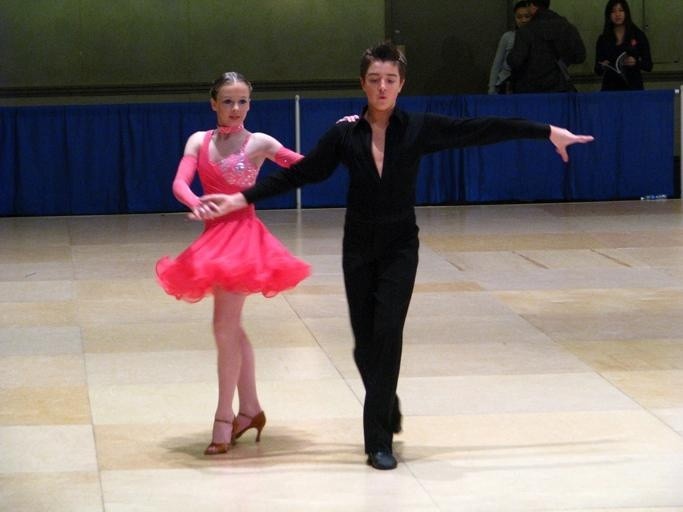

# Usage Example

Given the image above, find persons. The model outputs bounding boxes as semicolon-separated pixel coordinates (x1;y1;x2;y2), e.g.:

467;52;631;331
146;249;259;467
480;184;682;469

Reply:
487;1;530;97
496;0;586;94
186;37;598;471
592;0;654;91
153;70;364;455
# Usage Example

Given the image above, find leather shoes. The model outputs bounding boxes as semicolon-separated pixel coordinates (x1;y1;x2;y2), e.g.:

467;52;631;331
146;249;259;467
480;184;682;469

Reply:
370;450;396;469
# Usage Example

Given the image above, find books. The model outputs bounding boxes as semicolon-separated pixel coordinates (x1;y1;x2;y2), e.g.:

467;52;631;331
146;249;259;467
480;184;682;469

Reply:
598;51;628;74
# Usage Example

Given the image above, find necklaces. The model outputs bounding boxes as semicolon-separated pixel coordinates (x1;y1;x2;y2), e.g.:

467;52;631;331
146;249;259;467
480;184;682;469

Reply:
217;124;243;136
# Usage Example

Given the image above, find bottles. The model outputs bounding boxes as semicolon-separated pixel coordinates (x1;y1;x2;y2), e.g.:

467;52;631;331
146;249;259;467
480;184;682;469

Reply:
640;194;667;200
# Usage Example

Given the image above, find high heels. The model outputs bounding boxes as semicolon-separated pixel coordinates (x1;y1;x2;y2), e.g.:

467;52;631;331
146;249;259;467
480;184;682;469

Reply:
205;415;238;454
233;412;265;442
